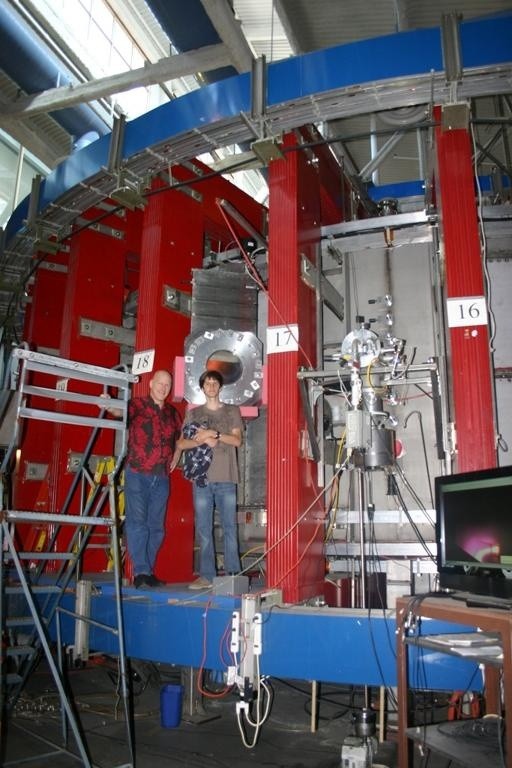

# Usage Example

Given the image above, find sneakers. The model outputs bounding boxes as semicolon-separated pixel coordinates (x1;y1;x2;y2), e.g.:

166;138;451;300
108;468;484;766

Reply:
189;577;213;590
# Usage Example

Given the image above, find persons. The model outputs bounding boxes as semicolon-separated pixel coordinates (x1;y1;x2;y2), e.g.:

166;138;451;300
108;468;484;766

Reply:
177;369;243;591
98;371;185;591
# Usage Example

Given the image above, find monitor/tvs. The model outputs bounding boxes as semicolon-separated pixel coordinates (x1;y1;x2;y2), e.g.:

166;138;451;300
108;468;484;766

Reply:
433;465;512;610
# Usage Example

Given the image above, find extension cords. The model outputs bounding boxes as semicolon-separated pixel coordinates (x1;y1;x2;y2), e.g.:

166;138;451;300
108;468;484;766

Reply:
231;612;239;652
252;613;261;654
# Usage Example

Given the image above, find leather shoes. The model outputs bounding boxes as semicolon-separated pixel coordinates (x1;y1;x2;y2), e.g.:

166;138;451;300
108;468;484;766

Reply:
135;575;152;589
148;574;167;587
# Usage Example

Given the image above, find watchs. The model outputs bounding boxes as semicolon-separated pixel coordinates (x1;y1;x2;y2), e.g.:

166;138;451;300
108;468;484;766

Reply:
214;429;222;440
